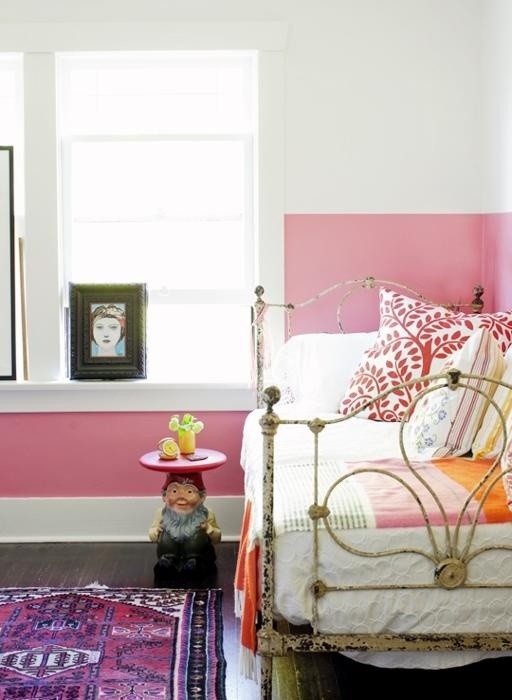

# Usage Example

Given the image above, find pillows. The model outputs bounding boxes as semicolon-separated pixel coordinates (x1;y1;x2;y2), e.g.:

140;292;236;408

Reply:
278;331;381;415
338;285;511;421
403;327;507;462
467;344;510;460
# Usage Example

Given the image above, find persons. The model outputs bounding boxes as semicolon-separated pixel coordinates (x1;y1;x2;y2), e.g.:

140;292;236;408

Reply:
148;473;222;589
90;305;125;357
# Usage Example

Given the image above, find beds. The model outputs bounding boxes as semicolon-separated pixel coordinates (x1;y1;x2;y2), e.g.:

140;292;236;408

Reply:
232;272;511;700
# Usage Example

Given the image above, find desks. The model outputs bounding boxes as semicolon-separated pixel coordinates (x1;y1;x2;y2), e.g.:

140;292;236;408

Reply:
137;446;228;578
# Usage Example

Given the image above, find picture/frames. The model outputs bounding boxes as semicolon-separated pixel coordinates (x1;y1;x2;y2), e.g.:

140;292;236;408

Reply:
65;280;148;383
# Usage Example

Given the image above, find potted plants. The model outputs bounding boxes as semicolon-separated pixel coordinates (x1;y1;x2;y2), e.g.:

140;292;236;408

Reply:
168;412;204;454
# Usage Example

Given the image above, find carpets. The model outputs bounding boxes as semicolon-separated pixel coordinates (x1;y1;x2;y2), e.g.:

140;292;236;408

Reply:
0;583;227;700
